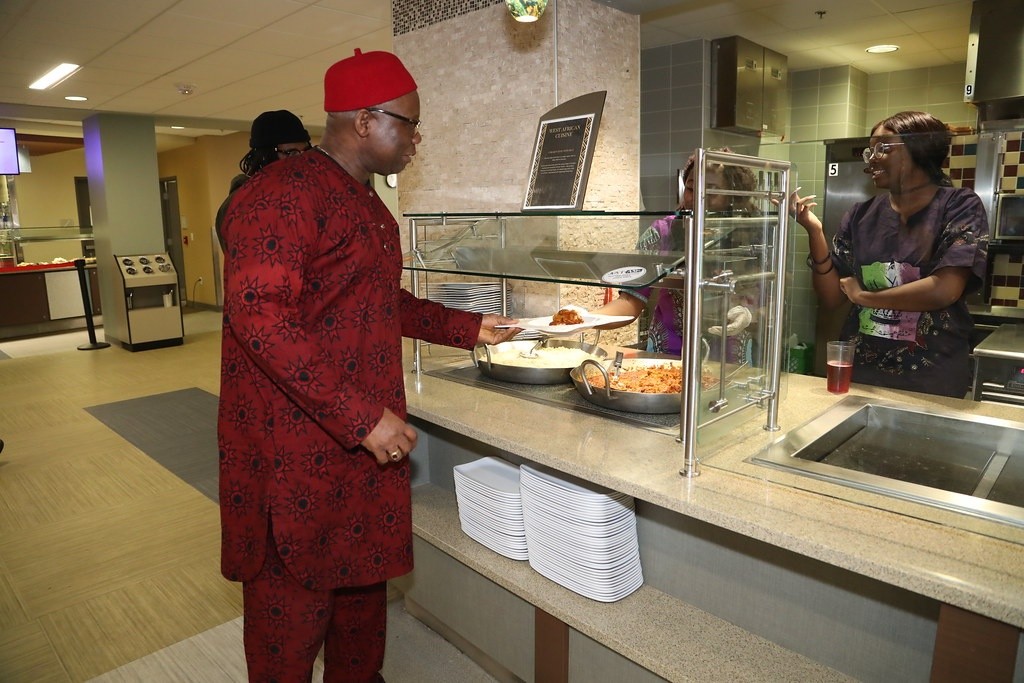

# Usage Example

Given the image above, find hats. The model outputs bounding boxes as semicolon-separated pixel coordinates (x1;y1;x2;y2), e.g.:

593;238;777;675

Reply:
250;110;311;149
323;48;417;113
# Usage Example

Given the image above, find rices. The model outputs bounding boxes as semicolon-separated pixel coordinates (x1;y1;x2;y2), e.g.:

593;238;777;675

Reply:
479;346;595;367
557;304;592;323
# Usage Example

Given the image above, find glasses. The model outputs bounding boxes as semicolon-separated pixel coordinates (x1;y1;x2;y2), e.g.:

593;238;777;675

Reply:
862;142;906;164
365;108;422;138
274;147;308;159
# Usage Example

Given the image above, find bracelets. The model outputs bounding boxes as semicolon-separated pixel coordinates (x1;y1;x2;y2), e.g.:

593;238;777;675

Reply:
806;253;834;274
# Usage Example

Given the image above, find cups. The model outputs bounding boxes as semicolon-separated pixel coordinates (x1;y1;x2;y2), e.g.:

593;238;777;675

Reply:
827;341;856;394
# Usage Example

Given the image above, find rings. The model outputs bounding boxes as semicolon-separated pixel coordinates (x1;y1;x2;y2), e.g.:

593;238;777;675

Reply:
392;449;401;460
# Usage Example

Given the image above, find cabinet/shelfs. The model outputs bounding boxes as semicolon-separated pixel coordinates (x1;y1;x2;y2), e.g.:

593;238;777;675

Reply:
712;34;788;139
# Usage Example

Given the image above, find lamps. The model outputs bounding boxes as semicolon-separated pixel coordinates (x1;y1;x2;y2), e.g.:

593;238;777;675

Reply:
505;0;548;22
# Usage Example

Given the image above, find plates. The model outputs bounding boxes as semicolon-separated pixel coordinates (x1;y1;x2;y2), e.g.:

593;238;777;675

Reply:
421;282;513;318
494;315;634;335
453;455;645;602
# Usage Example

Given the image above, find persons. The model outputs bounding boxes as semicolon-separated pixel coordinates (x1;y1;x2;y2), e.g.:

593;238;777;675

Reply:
771;111;989;399
589;147;774;366
217;48;526;683
215;110;313;251
